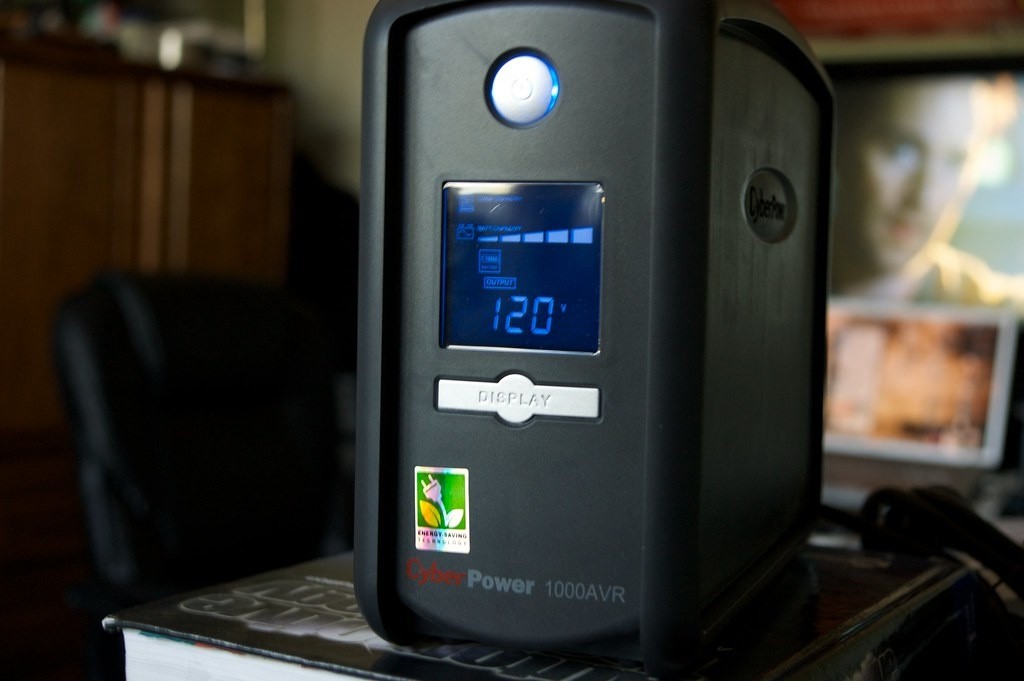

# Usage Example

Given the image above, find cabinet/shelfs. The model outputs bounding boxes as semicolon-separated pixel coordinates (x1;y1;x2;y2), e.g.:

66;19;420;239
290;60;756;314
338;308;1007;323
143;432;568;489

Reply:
0;14;295;681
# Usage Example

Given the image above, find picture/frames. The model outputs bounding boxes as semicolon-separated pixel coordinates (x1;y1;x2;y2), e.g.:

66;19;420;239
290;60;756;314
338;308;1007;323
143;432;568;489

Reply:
822;295;1018;469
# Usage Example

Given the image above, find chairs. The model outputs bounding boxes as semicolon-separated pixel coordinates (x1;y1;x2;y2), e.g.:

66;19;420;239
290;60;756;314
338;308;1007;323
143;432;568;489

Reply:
62;270;335;602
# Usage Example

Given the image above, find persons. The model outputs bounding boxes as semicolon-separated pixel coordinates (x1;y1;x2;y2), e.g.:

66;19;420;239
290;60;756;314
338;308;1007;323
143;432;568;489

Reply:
833;78;1024;308
